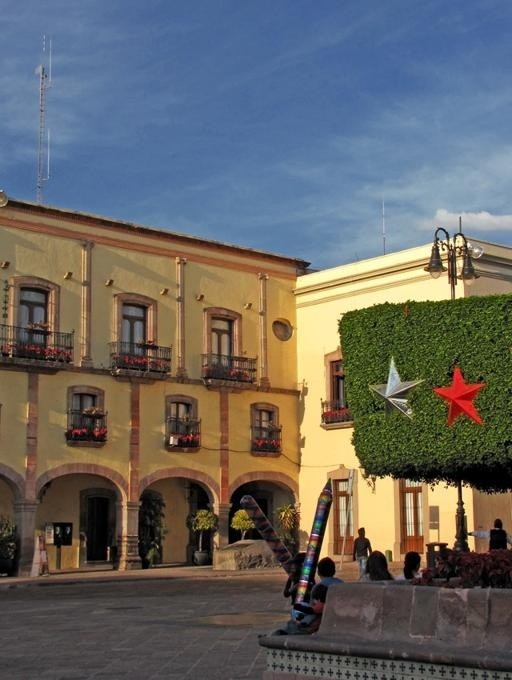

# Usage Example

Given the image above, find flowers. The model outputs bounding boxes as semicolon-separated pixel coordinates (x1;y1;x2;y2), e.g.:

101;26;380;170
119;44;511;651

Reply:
254;436;281;449
110;353;170;373
170;431;200;444
320;408;350;421
200;362;257;381
5;340;72;363
64;422;107;440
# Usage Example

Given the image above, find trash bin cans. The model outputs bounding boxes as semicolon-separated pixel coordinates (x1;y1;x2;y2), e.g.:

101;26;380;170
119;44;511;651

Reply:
107;546;116;562
426;542;448;568
386;550;392;561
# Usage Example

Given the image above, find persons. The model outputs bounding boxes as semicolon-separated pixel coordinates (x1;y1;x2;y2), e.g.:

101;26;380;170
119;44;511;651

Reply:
397;552;421;580
466;518;512;551
360;549;395;581
351;526;373;581
282;552;345;636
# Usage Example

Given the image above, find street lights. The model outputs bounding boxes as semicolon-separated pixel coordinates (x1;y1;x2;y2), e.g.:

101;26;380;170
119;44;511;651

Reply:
421;223;487;552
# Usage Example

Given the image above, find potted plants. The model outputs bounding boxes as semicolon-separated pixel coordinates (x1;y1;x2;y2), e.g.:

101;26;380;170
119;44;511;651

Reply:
190;507;218;565
